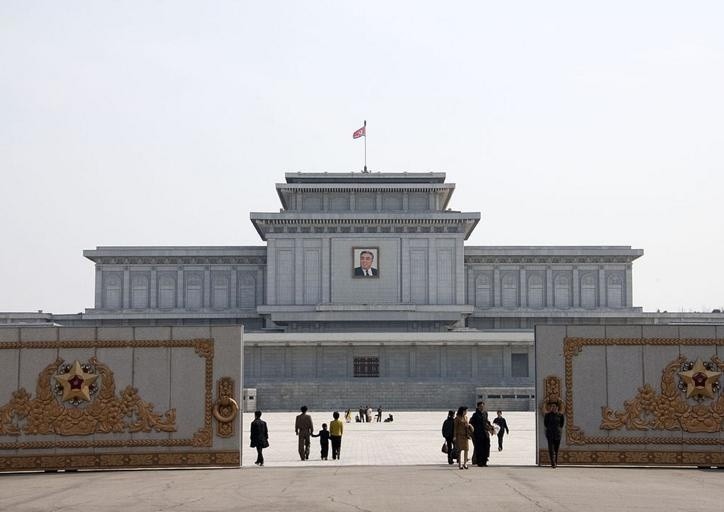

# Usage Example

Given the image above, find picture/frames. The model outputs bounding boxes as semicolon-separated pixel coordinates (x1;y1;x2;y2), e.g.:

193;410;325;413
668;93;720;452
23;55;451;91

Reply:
350;246;380;280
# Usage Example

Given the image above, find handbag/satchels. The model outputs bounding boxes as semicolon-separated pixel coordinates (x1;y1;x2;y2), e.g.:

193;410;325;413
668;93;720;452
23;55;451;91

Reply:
450;448;460;459
442;443;449;452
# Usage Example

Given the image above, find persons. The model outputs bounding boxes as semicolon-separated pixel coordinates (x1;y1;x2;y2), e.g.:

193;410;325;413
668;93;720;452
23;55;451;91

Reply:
355;251;377;277
295;406;313;460
250;411;269;466
544;402;564;468
312;424;332;460
330;412;343;459
442;402;508;469
353;127;365;139
345;405;393;423
